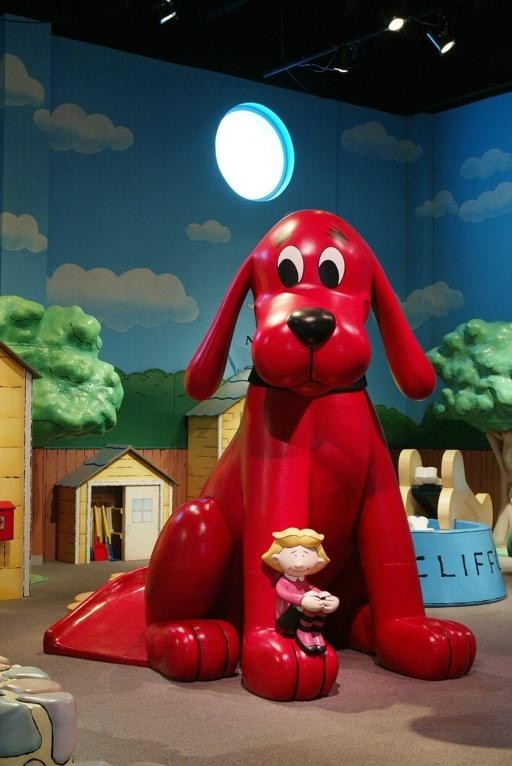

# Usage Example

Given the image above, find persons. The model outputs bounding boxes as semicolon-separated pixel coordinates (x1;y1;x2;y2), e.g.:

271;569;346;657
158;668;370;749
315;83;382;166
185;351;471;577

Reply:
260;526;339;655
